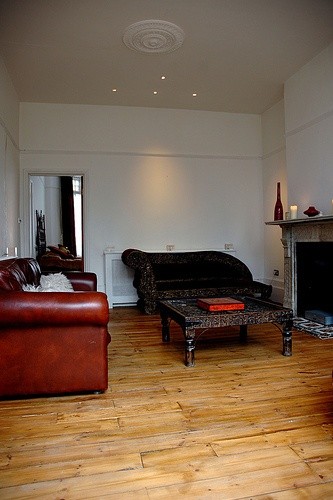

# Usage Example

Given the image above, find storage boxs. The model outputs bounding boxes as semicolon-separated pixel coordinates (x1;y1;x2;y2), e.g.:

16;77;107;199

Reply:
196;297;244;312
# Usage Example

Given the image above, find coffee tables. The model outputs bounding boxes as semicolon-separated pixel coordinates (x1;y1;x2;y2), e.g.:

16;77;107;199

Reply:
159;295;293;368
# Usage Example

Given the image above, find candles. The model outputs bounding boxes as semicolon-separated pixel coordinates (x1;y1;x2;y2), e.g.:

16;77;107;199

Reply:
290;205;298;219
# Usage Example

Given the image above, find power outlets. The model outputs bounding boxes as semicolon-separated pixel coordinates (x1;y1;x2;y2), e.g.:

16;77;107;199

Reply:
274;270;279;276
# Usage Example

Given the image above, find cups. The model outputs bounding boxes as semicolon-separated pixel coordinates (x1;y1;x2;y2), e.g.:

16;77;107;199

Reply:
290;205;298;220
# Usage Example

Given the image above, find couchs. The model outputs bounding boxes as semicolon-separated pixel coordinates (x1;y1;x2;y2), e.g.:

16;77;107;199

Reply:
120;248;272;315
0;243;111;400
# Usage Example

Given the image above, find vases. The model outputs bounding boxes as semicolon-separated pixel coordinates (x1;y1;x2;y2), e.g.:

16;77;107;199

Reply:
303;206;321;217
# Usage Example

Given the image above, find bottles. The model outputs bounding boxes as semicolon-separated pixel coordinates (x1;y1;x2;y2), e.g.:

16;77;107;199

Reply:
274;182;283;221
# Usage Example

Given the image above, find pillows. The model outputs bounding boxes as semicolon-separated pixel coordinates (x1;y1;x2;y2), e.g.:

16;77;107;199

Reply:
39;272;74;291
